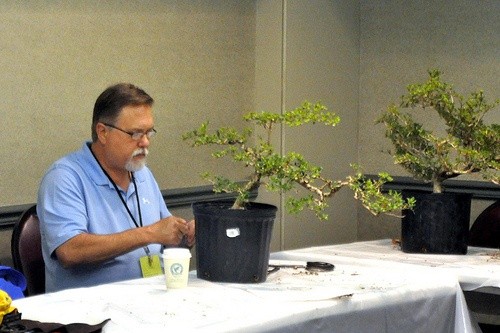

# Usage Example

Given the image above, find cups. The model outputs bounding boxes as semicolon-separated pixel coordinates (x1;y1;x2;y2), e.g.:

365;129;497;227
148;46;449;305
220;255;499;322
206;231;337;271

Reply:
160;248;192;289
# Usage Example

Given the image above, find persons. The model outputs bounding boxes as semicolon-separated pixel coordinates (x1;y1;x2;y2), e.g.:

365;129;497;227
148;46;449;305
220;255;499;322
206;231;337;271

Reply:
36;83;196;293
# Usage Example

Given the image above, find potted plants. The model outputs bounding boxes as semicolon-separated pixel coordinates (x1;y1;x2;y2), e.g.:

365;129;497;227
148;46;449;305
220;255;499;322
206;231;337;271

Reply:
182;98;419;283
374;66;500;255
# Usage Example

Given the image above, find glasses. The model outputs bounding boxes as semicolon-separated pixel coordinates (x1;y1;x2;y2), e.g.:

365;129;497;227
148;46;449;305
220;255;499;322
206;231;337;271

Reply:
103;122;157;141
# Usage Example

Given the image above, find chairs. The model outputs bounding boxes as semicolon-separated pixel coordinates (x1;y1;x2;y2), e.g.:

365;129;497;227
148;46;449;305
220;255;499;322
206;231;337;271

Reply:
10;204;46;298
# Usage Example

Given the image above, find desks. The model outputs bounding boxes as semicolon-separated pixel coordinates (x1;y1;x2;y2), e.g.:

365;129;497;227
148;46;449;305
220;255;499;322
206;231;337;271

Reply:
11;238;500;333
